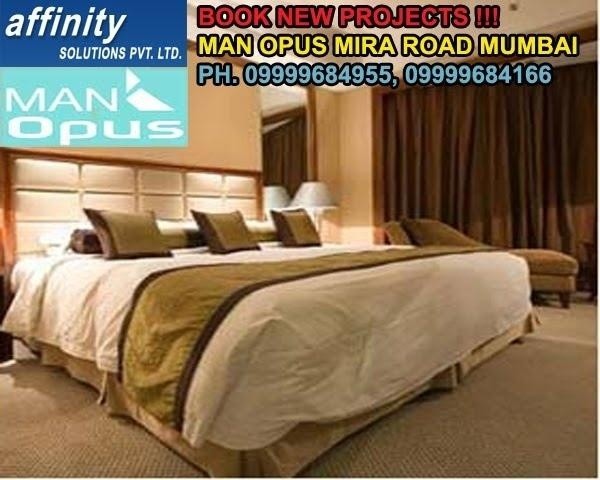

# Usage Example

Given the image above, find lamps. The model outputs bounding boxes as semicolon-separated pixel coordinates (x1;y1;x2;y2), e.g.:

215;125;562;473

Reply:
262;185;291;221
288;179;337;228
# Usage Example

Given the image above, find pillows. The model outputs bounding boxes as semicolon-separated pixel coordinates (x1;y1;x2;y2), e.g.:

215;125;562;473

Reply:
83;205;172;260
190;208;261;254
70;222;281;254
272;209;322;247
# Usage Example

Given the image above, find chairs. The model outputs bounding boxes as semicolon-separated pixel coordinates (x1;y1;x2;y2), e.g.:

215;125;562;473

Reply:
373;217;579;310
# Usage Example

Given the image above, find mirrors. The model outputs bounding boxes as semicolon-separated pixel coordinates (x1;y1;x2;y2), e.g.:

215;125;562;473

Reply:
258;79;321;222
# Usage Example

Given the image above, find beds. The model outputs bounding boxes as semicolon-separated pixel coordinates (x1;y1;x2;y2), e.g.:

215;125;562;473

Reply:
0;148;548;476
